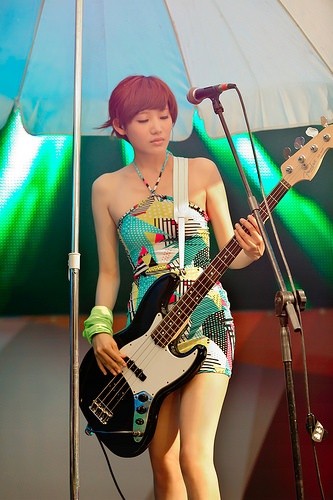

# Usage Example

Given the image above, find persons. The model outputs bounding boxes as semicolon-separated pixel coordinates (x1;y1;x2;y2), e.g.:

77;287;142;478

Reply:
80;75;266;500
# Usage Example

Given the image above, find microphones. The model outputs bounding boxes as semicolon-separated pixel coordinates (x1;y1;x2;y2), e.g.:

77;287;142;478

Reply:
186;83;236;105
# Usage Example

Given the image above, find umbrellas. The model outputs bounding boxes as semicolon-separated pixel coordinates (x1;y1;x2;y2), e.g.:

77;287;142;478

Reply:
1;0;333;500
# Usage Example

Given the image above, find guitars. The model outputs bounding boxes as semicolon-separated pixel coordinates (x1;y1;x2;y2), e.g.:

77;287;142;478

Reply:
78;115;333;458
